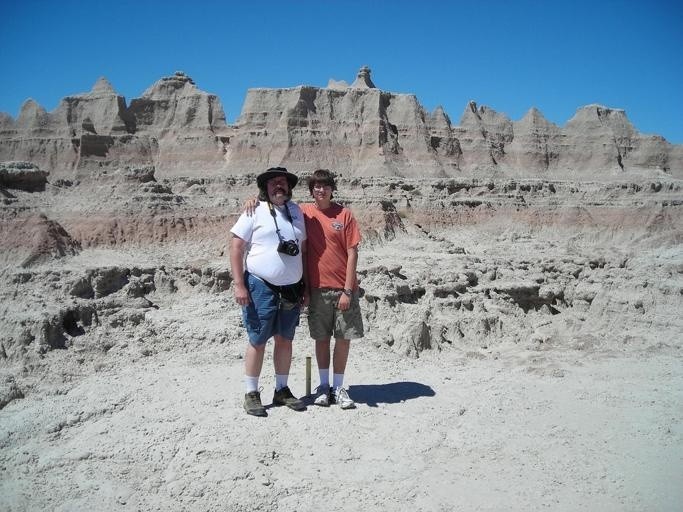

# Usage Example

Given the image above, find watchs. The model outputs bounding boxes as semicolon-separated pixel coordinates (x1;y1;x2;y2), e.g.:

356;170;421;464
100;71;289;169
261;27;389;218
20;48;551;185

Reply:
342;287;353;295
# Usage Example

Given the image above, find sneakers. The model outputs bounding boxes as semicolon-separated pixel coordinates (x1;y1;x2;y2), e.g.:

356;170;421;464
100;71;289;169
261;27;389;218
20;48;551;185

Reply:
243;391;266;415
273;385;306;410
313;384;330;406
330;386;355;409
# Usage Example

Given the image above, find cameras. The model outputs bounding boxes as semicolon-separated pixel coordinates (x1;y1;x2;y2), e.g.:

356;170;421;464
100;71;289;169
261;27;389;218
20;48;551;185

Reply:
277;239;300;255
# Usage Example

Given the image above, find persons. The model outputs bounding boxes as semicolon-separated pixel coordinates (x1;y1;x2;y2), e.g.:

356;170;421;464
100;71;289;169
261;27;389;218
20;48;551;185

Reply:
242;168;363;410
228;168;310;415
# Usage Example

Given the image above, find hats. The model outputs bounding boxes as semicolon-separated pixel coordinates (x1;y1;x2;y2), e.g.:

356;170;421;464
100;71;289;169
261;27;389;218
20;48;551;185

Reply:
257;167;298;191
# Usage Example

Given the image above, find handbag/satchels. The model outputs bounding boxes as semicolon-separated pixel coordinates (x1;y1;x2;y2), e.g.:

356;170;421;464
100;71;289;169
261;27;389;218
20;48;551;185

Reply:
258;274;306;304
